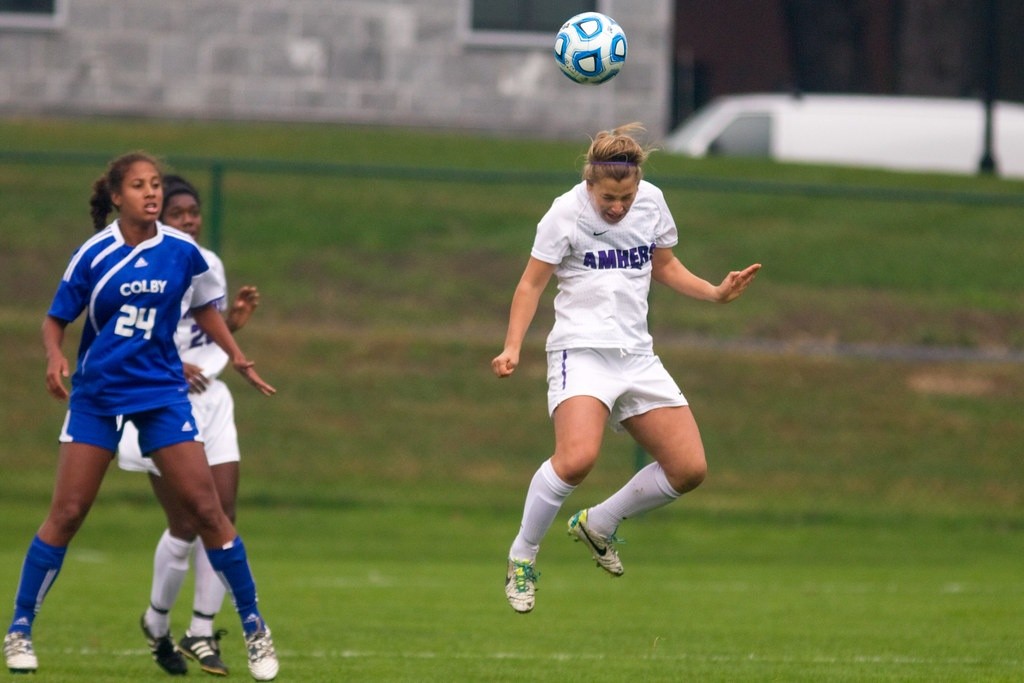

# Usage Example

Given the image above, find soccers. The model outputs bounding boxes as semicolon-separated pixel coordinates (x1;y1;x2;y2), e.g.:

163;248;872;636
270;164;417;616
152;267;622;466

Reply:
555;12;628;86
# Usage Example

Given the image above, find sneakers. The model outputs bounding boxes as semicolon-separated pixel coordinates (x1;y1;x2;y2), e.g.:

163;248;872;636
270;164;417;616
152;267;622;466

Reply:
177;631;228;676
140;614;188;675
3;631;39;673
506;556;536;612
239;621;280;680
567;507;625;575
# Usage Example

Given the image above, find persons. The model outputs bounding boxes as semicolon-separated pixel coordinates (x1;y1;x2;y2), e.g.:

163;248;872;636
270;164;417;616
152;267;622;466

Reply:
492;119;762;613
2;152;280;681
140;173;261;678
